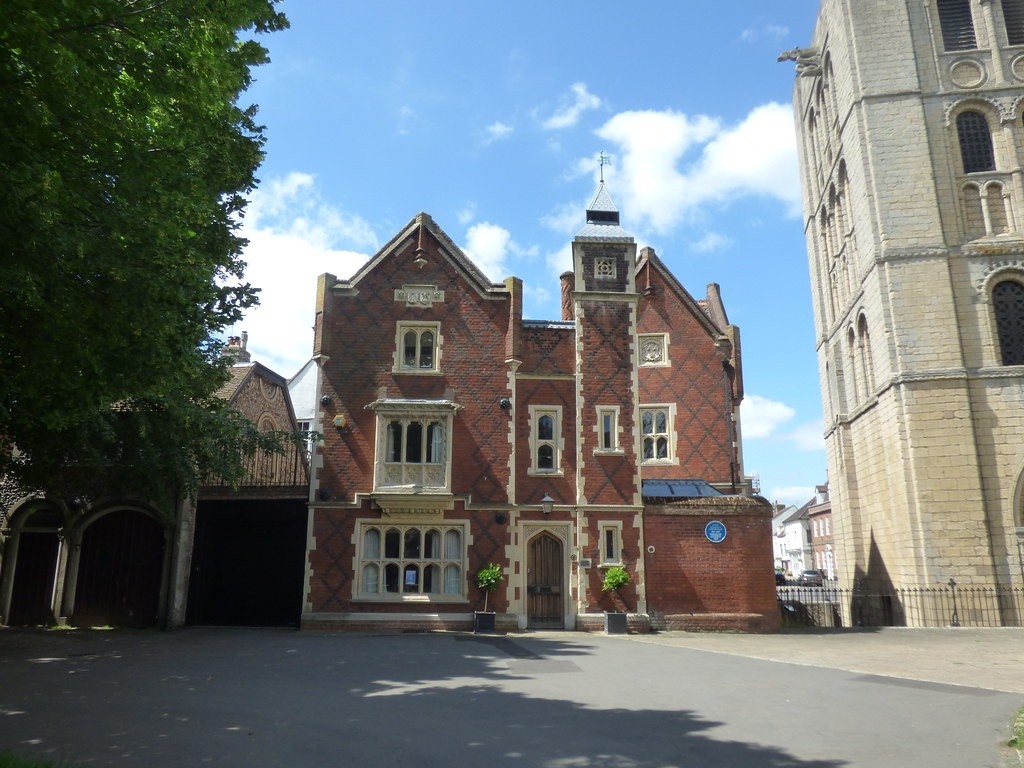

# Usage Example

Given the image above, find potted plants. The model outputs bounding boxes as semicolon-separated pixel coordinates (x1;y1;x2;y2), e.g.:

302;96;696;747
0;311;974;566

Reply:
471;561;504;632
600;566;631;635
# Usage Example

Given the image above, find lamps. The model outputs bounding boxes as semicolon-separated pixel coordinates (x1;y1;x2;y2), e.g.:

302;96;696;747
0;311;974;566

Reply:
540;492;555;517
333;415;347;429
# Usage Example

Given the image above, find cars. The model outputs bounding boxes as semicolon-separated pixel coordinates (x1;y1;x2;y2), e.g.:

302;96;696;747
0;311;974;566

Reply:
797;571;823;588
775;569;788;586
